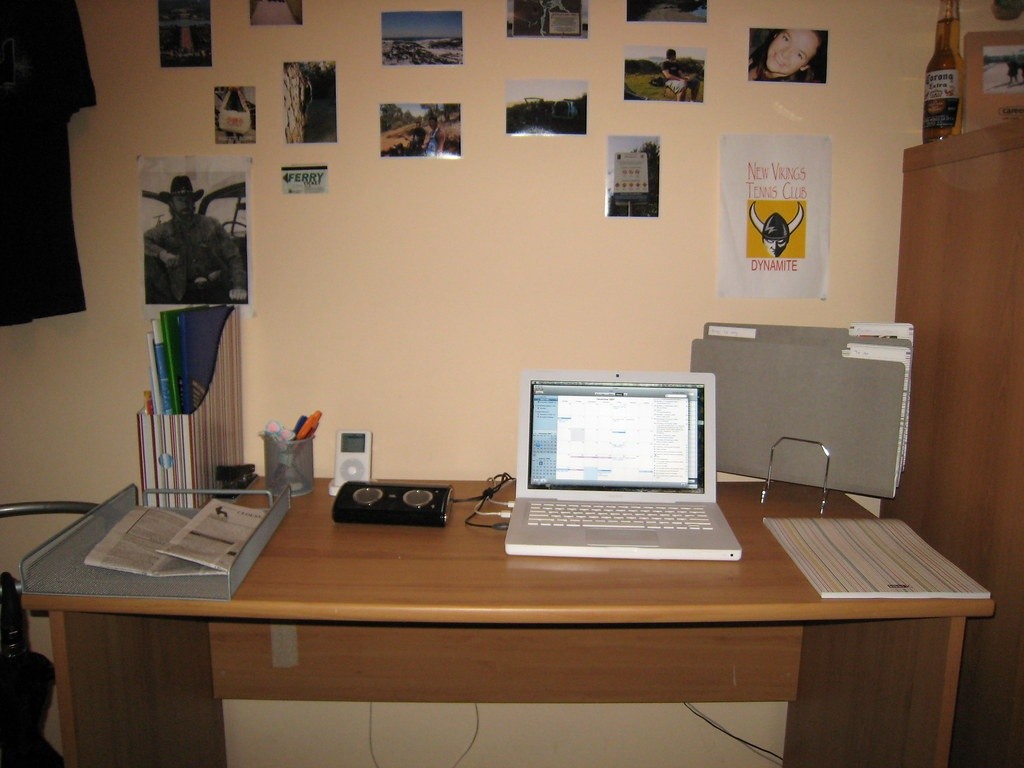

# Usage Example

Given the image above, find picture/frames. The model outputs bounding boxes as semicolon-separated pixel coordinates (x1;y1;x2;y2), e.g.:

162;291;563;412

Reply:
963;30;1024;134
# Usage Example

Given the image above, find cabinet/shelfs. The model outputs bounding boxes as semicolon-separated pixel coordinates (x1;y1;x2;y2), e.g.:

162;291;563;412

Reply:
879;117;1024;768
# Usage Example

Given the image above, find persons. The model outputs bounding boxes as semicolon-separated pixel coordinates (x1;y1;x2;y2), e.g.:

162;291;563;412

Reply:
748;28;821;82
412;115;445;157
142;175;248;310
661;48;700;102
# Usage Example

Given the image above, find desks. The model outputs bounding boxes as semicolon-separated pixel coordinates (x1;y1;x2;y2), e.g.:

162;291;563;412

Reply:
22;478;995;768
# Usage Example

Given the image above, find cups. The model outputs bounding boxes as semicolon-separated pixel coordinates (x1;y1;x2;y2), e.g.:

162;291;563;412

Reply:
264;440;314;495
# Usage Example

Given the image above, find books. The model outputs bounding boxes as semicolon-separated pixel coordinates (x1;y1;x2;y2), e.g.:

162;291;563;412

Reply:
143;304;234;416
762;517;991;599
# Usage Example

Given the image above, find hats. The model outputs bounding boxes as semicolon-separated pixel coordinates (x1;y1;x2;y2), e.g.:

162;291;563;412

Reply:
158;175;205;203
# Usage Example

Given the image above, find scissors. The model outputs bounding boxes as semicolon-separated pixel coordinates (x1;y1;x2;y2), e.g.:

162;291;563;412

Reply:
257;410;323;497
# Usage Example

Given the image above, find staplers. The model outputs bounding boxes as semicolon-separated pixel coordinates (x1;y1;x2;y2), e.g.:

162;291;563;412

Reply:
211;463;259;504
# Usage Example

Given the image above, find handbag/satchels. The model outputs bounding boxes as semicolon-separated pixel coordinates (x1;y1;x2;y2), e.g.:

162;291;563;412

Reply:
0;570;64;768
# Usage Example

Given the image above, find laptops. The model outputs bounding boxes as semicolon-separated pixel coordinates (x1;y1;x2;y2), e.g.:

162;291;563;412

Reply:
505;369;743;561
